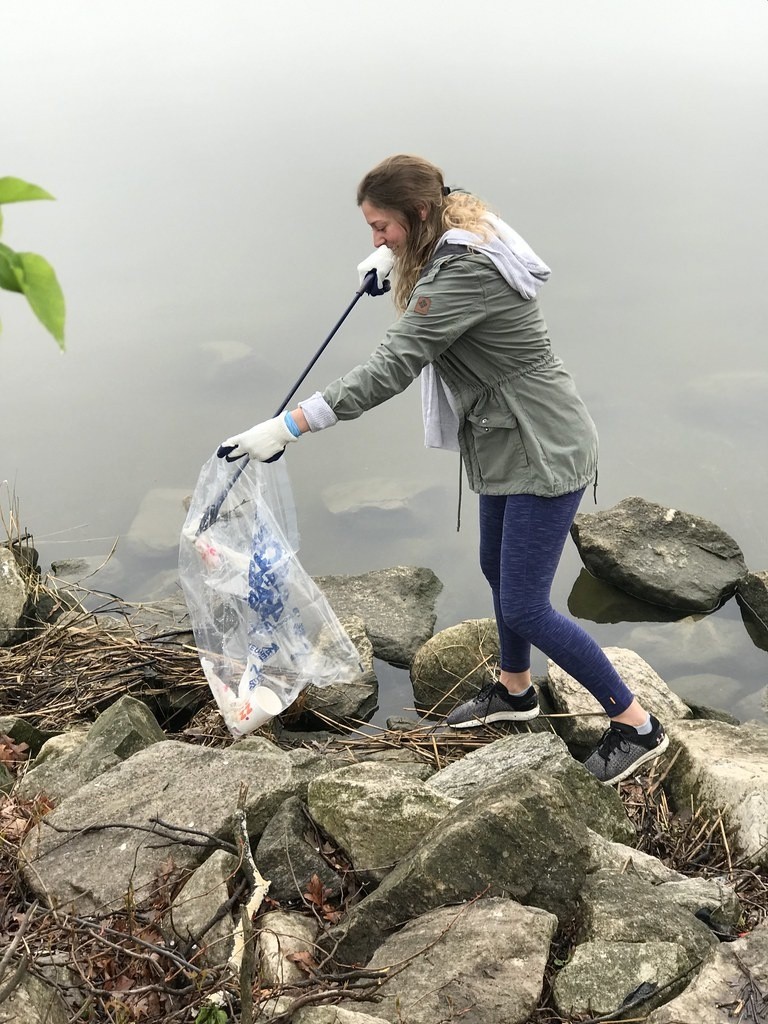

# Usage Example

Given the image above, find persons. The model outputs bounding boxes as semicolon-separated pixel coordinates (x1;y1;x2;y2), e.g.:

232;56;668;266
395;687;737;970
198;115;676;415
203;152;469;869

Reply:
217;154;669;788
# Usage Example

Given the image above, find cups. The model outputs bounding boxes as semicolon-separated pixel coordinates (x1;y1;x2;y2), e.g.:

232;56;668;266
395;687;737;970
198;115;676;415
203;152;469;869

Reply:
233;685;283;734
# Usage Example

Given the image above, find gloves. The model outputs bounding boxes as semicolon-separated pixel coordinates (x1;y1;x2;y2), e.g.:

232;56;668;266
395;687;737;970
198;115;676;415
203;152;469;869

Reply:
217;411;301;463
357;245;397;297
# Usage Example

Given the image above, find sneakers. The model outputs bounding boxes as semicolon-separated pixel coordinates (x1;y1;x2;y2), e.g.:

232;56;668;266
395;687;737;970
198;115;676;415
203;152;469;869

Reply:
446;682;540;728
584;714;670;786
